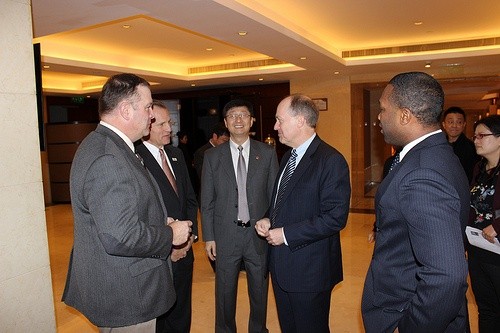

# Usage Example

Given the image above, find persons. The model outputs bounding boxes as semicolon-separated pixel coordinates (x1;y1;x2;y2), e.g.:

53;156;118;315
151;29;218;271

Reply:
134;99;198;333
360;72;469;333
367;145;403;243
442;107;481;185
464;115;500;333
176;131;191;177
255;94;350;333
201;100;279;333
61;74;192;333
194;124;231;271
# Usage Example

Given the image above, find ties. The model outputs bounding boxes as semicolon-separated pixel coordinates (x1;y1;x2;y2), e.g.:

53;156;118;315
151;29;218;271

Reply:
134;154;144;167
272;149;298;227
388;151;400;171
159;148;179;193
236;145;252;224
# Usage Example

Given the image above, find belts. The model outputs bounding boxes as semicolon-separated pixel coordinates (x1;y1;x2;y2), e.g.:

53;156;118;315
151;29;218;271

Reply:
237;220;250;227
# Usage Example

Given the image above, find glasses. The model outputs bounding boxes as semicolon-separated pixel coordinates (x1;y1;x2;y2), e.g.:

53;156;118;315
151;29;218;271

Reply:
473;133;495;140
224;113;251;120
